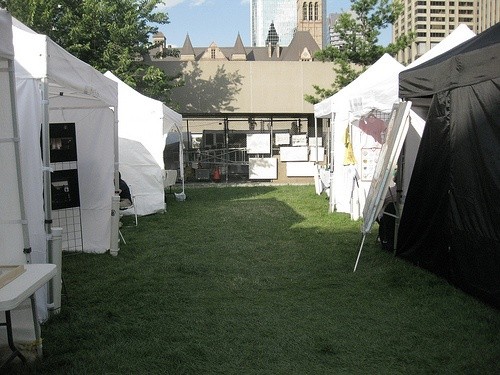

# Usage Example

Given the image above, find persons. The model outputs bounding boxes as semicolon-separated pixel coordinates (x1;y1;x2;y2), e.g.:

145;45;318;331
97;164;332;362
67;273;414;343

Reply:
113;170;133;227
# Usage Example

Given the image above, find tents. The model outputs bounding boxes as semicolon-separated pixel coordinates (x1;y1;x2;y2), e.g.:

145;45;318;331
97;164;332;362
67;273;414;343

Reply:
102;70;186;216
314;52;406;213
403;24;477;72
12;16;122;315
0;6;43;366
379;22;500;313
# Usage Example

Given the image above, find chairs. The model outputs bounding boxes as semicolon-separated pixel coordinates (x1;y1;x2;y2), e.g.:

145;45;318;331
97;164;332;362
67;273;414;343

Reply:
119;184;137;226
166;170;177;194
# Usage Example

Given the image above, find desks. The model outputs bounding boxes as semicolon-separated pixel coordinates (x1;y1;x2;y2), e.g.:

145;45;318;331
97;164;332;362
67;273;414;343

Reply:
0;264;57;375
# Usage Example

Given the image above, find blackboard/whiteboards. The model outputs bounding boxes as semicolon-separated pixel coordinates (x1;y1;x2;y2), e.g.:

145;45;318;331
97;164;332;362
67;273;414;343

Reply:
246;133;325;181
